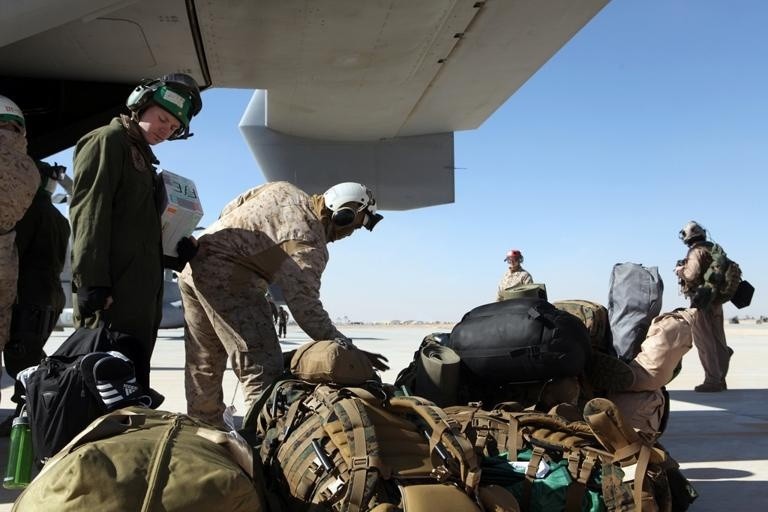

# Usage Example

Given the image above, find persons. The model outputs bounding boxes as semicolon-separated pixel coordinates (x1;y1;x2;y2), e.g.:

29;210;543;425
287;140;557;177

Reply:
5;160;72;413
672;220;755;390
72;75;202;384
178;181;389;430
278;307;289;338
496;251;534;302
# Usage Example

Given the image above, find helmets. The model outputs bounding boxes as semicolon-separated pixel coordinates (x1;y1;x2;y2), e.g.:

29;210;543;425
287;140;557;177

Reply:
0;95;25;125
34;162;57;195
679;220;705;246
325;183;382;231
126;75;202;140
504;249;523;263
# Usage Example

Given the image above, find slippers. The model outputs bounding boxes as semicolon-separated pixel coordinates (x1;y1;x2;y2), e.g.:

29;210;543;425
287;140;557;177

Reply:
93;357;139;413
81;352;114;412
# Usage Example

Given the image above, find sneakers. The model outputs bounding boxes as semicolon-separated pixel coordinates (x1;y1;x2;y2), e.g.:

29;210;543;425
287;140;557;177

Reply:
695;380;726;392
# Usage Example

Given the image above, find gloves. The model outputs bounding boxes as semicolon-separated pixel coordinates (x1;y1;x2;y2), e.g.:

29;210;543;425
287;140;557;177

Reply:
77;288;112;316
175;236;199;262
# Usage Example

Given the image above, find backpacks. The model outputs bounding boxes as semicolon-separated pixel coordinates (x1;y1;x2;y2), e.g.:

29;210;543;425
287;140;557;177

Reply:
0;93;41;353
289;339;385;388
689;243;743;306
14;408;268;511
442;396;699;512
393;297;593;412
241;375;483;512
16;306;166;471
